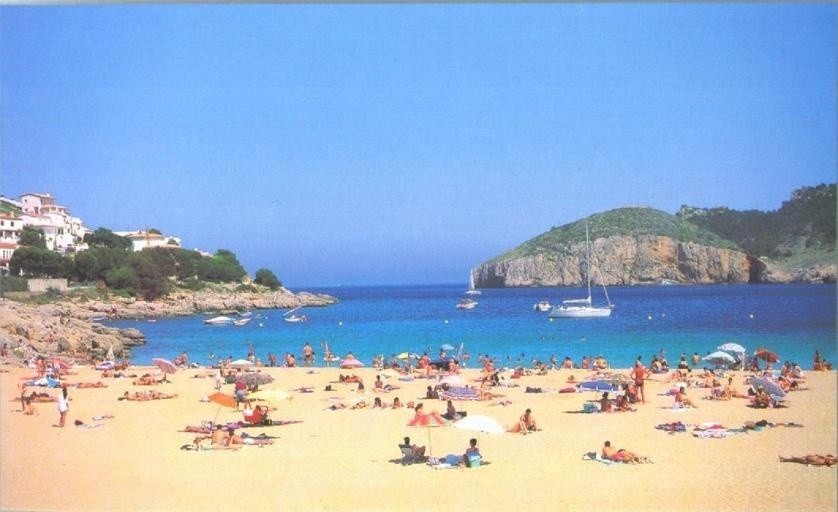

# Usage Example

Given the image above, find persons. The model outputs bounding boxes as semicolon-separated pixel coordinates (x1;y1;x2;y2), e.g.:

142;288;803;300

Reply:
104;304;117;314
0;338;837;468
59;315;72;328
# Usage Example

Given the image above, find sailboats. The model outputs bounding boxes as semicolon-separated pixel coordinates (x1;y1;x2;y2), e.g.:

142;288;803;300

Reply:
548;222;615;317
466;269;482;296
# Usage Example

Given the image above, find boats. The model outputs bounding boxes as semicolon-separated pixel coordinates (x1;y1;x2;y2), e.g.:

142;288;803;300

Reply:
532;301;550;312
455;297;477;309
286;315;307;323
204;316;249;326
